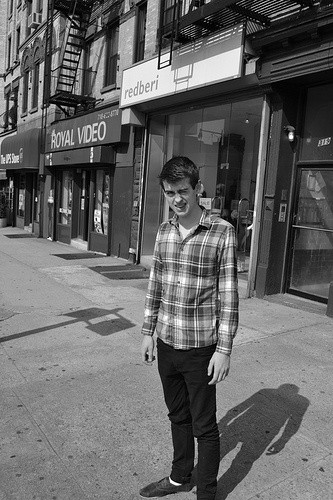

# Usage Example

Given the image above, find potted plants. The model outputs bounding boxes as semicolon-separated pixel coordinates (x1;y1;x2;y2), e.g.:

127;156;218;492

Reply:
0;194;7;227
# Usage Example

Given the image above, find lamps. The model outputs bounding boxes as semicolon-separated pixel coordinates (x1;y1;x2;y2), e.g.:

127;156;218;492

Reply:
284;126;295;142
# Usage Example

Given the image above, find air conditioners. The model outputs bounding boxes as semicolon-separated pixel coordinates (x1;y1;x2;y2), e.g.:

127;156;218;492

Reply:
28;13;42;28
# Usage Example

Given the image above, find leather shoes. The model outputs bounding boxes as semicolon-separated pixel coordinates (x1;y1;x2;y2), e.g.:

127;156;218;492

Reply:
139;475;192;497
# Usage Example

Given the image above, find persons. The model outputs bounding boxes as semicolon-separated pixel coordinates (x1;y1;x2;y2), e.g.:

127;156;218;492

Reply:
140;155;239;500
